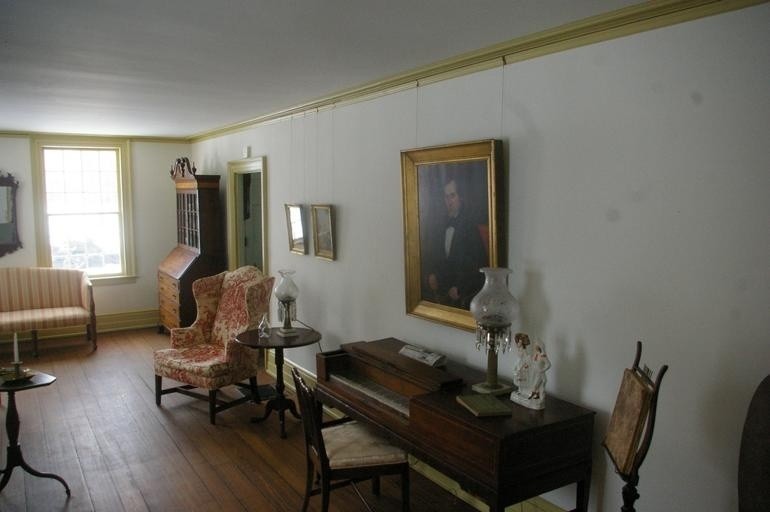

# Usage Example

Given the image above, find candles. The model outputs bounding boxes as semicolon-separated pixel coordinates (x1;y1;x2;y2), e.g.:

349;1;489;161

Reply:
13;332;19;362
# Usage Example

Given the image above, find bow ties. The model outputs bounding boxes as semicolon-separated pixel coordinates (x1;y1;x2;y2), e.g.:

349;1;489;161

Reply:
446;215;456;227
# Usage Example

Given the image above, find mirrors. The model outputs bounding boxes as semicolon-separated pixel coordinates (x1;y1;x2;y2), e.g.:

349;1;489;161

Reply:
0;169;23;257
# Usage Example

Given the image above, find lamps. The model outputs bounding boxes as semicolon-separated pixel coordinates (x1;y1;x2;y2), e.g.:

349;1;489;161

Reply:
470;267;520;396
274;268;299;338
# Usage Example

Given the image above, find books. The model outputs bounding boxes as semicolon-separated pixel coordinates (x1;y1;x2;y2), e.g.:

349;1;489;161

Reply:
604;368;656;476
456;393;512;417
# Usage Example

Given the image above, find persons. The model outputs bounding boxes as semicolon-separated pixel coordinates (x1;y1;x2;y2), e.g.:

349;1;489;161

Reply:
427;176;489;311
512;337;551;400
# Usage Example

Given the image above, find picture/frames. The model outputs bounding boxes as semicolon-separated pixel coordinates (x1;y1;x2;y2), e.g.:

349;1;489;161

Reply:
400;138;499;334
284;203;308;256
310;203;335;262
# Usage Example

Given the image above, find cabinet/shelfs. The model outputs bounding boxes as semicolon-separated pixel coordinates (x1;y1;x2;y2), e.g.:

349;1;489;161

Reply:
157;156;221;333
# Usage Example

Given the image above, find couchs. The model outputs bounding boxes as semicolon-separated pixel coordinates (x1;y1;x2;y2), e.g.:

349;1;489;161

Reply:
0;266;97;358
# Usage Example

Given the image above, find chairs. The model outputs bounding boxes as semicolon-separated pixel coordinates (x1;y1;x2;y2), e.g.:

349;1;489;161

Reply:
153;264;276;425
291;367;410;512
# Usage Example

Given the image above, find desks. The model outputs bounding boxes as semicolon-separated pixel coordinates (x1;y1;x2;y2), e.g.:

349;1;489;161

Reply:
0;365;71;498
235;327;321;439
